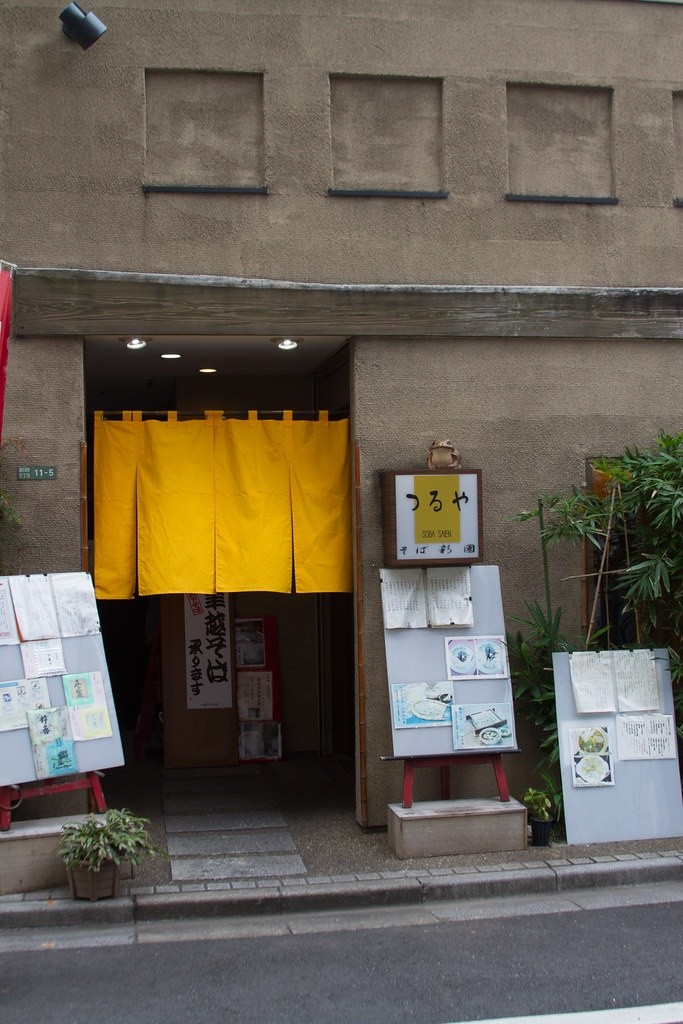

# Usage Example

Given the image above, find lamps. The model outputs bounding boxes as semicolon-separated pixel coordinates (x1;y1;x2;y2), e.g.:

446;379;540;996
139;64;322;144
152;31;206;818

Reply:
58;1;107;51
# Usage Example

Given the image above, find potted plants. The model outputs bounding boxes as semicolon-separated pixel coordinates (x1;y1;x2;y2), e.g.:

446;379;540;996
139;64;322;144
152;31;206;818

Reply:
525;787;554;845
59;809;170;899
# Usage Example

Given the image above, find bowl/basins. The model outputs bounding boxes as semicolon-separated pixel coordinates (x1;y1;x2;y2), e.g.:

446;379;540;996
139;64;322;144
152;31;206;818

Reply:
440;694;452;703
499;726;511;736
479;728;501;745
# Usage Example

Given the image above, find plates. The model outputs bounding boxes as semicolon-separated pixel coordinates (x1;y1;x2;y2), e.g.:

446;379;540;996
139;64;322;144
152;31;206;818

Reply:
577;728;608;755
450;644;476;674
476;642;503;675
576;755;608;782
